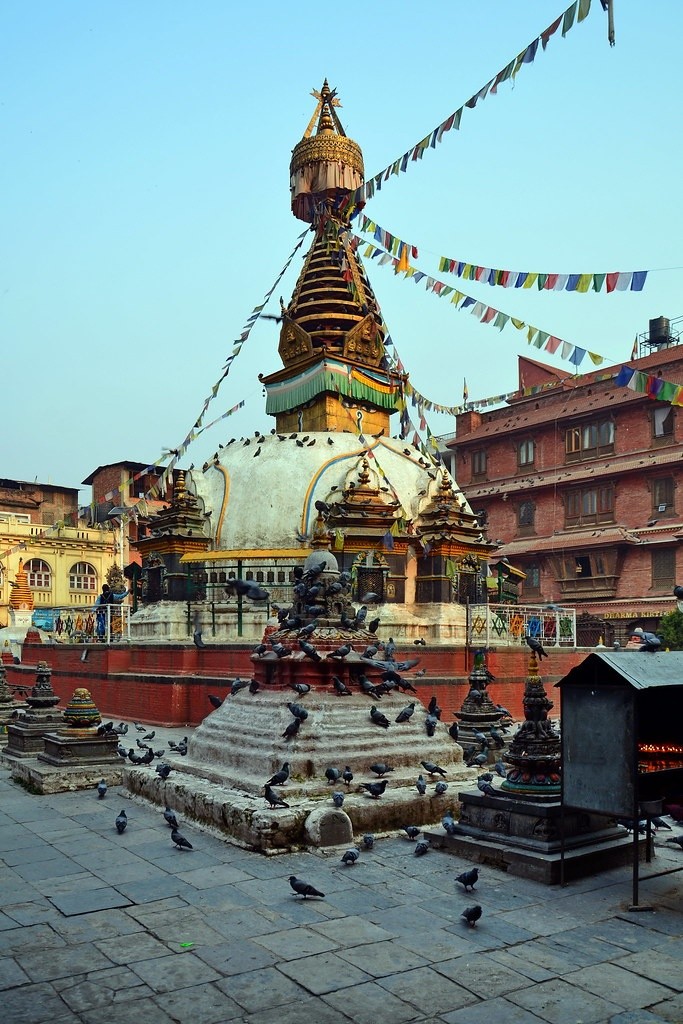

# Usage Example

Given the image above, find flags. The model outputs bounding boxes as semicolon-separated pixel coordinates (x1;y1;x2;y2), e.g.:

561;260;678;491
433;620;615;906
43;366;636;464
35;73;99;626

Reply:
631;338;638;360
464;382;468;399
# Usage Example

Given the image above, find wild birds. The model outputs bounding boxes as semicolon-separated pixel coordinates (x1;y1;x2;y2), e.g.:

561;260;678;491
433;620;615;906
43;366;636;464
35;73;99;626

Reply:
95;423;683;927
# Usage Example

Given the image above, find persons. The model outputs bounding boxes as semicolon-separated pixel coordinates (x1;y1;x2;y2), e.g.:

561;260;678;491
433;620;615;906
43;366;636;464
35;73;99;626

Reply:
92;584;131;641
